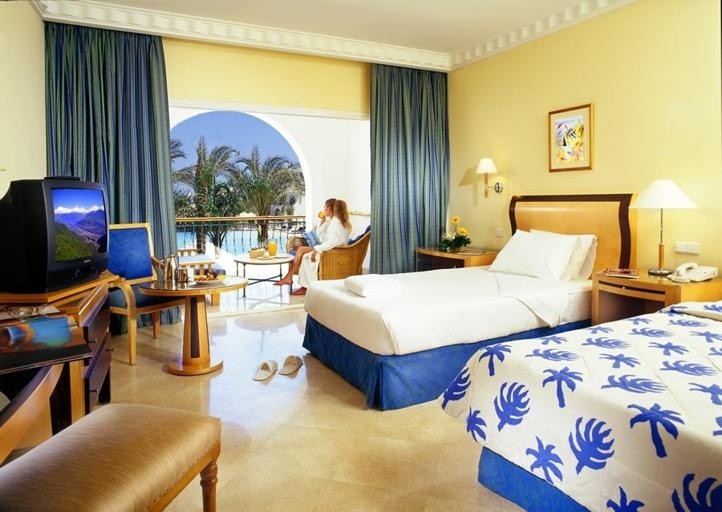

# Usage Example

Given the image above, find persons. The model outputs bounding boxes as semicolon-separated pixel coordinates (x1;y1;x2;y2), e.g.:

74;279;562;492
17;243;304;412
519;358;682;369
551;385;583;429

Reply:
274;199;350;295
290;198;336;274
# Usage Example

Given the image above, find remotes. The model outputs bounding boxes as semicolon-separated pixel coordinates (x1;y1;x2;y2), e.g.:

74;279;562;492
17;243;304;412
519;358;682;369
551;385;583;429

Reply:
38;175;86;182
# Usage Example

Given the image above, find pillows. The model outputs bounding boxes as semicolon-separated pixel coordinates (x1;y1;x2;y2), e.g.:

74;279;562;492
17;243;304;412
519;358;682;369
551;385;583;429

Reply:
488;227;597;284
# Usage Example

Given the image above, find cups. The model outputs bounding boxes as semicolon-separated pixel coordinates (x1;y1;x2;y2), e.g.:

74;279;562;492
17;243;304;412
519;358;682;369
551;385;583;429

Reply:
318;210;326;219
264;238;278;256
177;267;188;282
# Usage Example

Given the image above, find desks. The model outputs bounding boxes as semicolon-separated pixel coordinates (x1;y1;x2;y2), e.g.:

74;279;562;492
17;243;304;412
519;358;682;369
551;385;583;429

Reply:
0;362;64;468
415;245;501;272
591;270;722;326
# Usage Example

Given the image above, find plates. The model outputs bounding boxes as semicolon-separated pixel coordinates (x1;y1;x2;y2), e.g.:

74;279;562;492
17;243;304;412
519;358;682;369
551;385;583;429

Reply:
194;279;223;284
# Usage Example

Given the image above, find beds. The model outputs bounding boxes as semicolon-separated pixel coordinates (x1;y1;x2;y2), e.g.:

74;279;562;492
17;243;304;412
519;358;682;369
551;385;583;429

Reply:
304;192;638;411
476;301;722;512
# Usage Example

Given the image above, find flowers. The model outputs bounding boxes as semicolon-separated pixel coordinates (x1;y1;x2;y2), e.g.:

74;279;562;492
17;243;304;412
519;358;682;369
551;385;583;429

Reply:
440;215;472;253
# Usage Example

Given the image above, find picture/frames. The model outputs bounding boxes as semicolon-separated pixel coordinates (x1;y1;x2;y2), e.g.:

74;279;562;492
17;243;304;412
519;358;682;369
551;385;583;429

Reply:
547;102;594;173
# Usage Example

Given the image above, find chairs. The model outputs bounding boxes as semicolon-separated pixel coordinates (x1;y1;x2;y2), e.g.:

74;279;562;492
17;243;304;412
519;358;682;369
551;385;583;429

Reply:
286;228;373;280
106;222;185;365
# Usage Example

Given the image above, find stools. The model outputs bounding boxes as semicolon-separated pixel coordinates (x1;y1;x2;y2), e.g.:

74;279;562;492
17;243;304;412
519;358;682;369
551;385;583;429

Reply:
0;403;222;512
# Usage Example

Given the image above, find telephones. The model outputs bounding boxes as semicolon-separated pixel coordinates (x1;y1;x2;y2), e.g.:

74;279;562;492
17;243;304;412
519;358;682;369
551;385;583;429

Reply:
669;262;717;282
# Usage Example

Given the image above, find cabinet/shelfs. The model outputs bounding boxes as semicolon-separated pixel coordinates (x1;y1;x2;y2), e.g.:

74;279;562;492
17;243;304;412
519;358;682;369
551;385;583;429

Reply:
0;280;114;436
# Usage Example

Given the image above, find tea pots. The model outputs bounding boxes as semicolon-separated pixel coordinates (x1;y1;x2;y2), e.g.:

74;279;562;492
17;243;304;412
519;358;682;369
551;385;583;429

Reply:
158;255;178;282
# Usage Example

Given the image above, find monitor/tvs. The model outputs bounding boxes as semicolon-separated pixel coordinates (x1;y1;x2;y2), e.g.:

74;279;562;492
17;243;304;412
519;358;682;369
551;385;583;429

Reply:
0;179;111;294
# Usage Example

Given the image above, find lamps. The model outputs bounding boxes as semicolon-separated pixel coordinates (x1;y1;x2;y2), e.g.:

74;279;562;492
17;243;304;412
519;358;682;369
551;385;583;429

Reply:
627;177;696;277
475;157;504;199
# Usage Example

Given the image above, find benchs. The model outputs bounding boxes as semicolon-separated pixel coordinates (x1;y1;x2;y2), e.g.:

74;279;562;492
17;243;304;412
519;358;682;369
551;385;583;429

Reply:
176;248;226;307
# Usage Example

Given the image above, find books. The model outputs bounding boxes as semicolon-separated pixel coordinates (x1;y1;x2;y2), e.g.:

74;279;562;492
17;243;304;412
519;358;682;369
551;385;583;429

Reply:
603;267;640;279
0;305;94;376
457;249;484;255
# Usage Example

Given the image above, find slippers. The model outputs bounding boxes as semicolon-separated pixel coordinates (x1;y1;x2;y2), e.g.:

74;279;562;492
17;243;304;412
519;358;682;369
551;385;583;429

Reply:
253;359;278;381
278;355;302;375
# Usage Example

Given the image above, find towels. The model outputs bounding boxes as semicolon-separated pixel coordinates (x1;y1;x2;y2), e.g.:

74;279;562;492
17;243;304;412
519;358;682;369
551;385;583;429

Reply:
343;274;404;299
